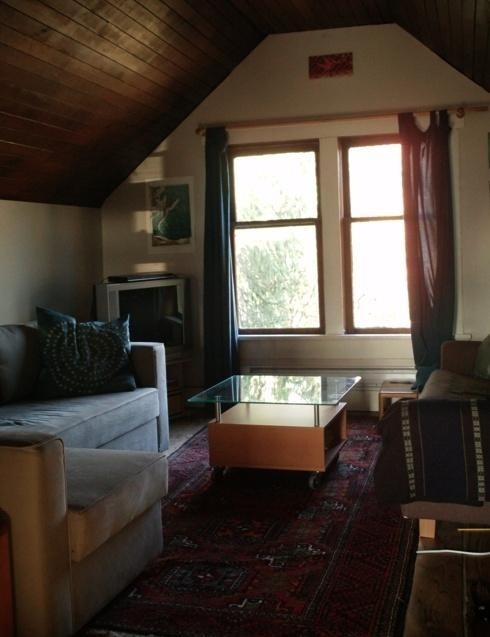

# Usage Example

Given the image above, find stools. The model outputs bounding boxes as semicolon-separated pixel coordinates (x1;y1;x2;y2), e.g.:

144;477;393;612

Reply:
378;380;418;421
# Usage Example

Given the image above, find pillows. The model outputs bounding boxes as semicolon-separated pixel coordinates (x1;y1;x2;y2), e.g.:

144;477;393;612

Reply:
16;305;138;397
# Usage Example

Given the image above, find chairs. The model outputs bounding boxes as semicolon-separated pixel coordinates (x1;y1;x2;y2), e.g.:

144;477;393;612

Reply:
392;335;490;541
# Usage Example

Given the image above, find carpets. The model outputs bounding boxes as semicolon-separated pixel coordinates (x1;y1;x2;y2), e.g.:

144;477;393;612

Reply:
83;407;418;636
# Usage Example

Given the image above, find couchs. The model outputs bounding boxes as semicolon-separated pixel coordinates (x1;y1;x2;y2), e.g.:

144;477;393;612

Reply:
1;313;172;637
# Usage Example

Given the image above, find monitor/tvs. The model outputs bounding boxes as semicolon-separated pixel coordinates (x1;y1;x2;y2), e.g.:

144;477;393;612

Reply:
92;279;187;363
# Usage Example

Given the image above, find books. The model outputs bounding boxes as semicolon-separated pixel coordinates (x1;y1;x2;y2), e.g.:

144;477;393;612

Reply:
108;274;176;283
